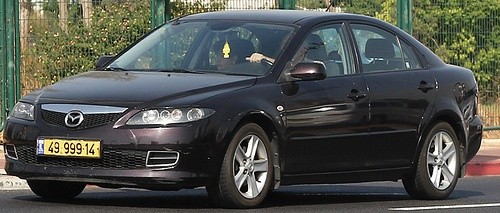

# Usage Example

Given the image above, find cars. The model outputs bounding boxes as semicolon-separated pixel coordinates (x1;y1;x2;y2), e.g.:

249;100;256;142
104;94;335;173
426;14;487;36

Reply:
3;10;483;209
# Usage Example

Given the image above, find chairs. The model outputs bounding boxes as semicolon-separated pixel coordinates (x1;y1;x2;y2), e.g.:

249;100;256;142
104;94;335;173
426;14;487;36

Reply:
230;39;255;64
306;33;342;76
361;38;403;71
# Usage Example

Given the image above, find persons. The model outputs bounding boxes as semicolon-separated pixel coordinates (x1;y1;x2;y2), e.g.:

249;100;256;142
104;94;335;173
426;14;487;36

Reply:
245;32;327;70
199;40;245;72
